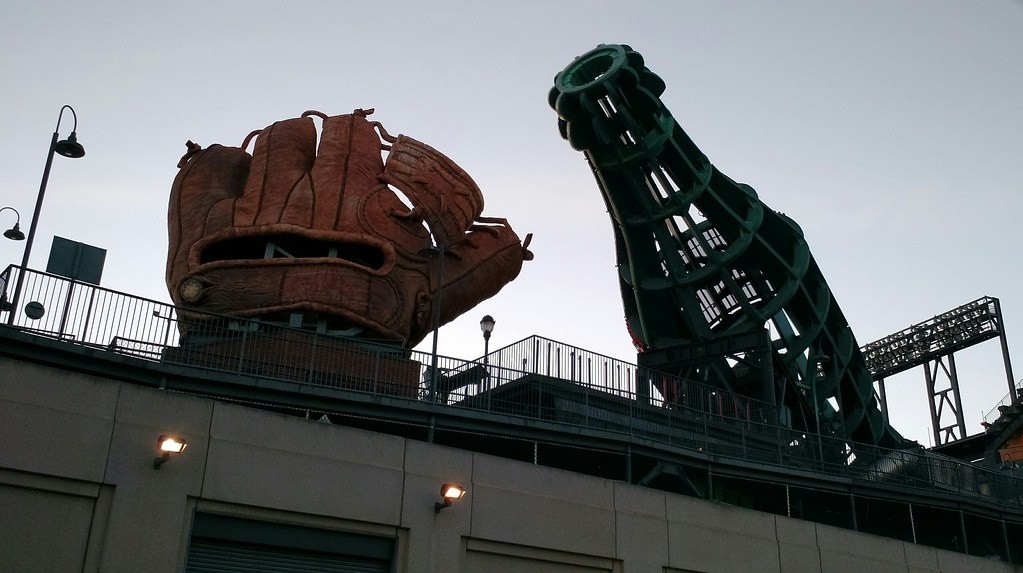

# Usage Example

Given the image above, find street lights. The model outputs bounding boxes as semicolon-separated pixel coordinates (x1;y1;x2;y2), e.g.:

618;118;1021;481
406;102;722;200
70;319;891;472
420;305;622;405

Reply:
7;104;85;326
480;315;495;397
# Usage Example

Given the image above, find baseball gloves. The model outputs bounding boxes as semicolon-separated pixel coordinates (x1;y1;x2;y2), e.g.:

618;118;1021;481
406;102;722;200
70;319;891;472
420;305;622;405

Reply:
163;110;536;400
